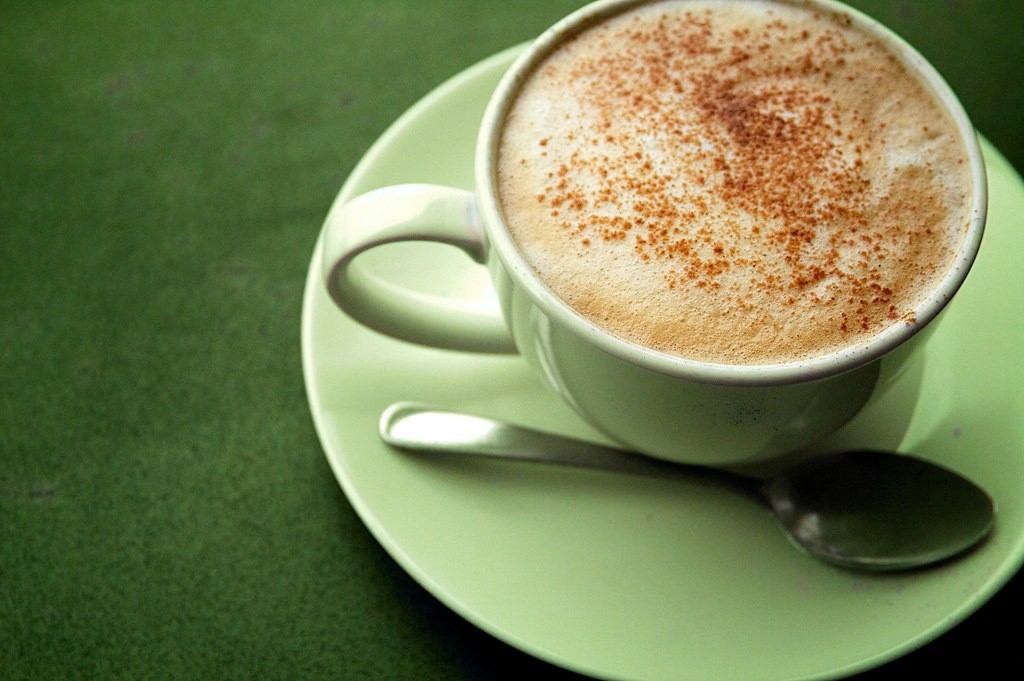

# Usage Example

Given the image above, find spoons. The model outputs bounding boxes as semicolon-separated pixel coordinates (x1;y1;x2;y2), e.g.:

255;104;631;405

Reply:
377;400;998;575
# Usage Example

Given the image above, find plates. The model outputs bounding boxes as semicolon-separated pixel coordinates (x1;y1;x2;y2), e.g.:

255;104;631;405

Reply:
300;38;1024;681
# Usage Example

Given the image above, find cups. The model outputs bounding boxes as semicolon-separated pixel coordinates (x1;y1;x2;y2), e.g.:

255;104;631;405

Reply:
320;4;990;468
